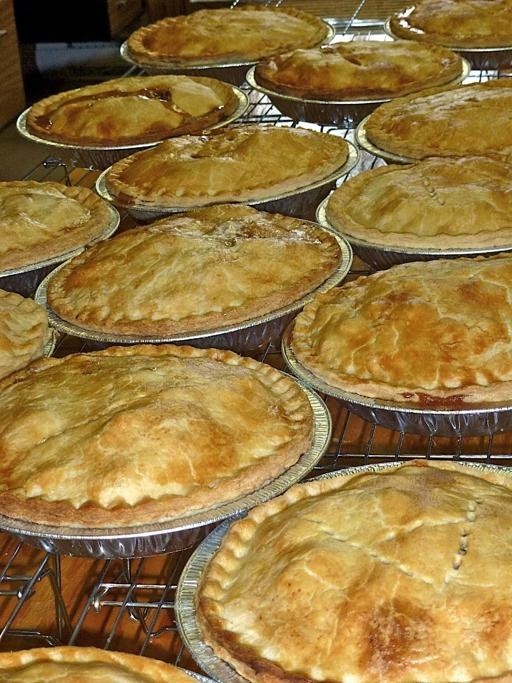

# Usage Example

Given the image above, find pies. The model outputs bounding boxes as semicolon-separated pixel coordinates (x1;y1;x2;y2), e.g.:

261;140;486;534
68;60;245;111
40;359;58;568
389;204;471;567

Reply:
0;181;111;272
2;290;54;379
389;1;510;49
193;460;511;682
0;646;199;683
364;78;510;160
289;252;512;403
325;156;512;249
26;76;238;147
47;204;342;338
1;344;315;528
253;41;463;102
106;125;351;207
126;5;329;67
